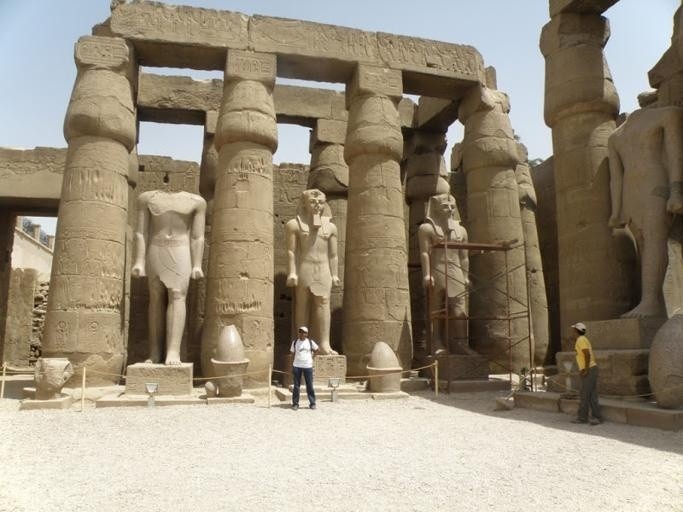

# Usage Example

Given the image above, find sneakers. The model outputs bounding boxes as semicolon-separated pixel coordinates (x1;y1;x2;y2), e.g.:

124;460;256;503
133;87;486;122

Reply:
292;404;299;409
310;404;315;408
571;418;601;424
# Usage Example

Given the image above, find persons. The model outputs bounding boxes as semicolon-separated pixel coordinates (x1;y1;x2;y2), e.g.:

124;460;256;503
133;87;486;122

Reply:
289;325;321;411
129;189;208;364
417;193;479;355
286;188;341;355
570;323;604;424
607;87;683;318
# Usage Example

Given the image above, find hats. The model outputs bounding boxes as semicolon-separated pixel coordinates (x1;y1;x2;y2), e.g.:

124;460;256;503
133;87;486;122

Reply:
571;323;586;332
299;327;308;333
